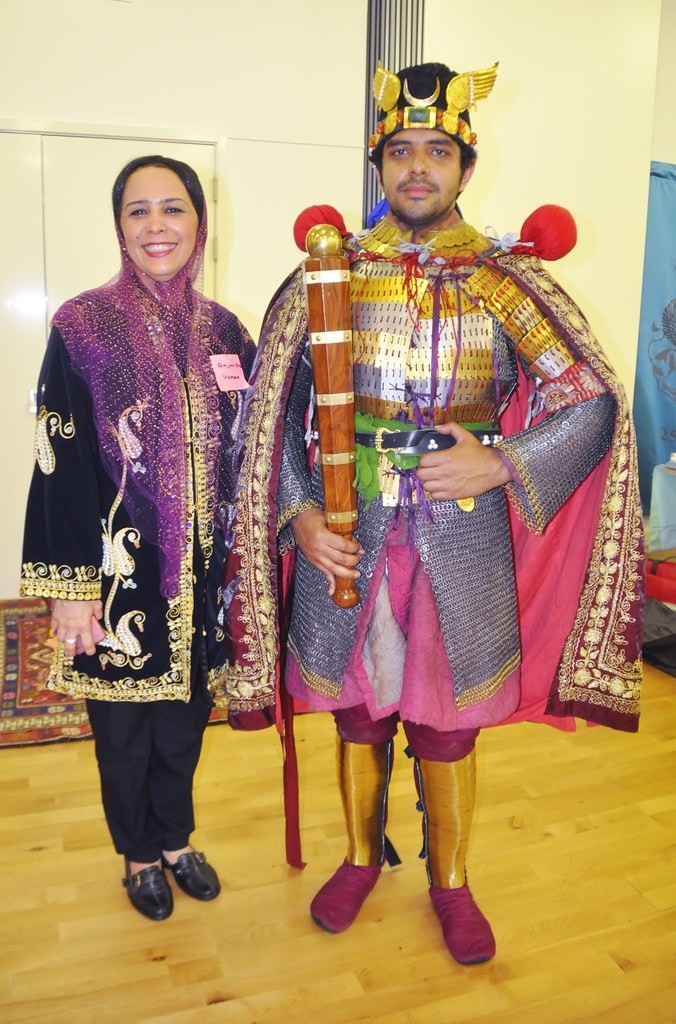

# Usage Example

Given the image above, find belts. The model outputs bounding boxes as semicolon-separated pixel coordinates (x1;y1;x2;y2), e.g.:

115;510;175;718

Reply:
355;426;502;454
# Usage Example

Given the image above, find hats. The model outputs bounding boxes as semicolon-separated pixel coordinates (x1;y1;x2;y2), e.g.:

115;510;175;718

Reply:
368;60;500;165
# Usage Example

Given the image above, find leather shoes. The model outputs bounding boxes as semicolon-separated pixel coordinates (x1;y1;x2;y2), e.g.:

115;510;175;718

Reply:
122;853;174;921
160;848;220;901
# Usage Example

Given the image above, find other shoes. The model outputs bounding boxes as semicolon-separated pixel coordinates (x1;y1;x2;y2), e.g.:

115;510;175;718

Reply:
310;856;383;934
429;881;496;965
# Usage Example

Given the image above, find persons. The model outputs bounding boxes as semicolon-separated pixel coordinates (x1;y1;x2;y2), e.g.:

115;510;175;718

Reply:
224;63;643;966
20;156;261;921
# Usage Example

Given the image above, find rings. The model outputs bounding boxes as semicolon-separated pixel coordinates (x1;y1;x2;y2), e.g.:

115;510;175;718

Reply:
60;636;78;646
